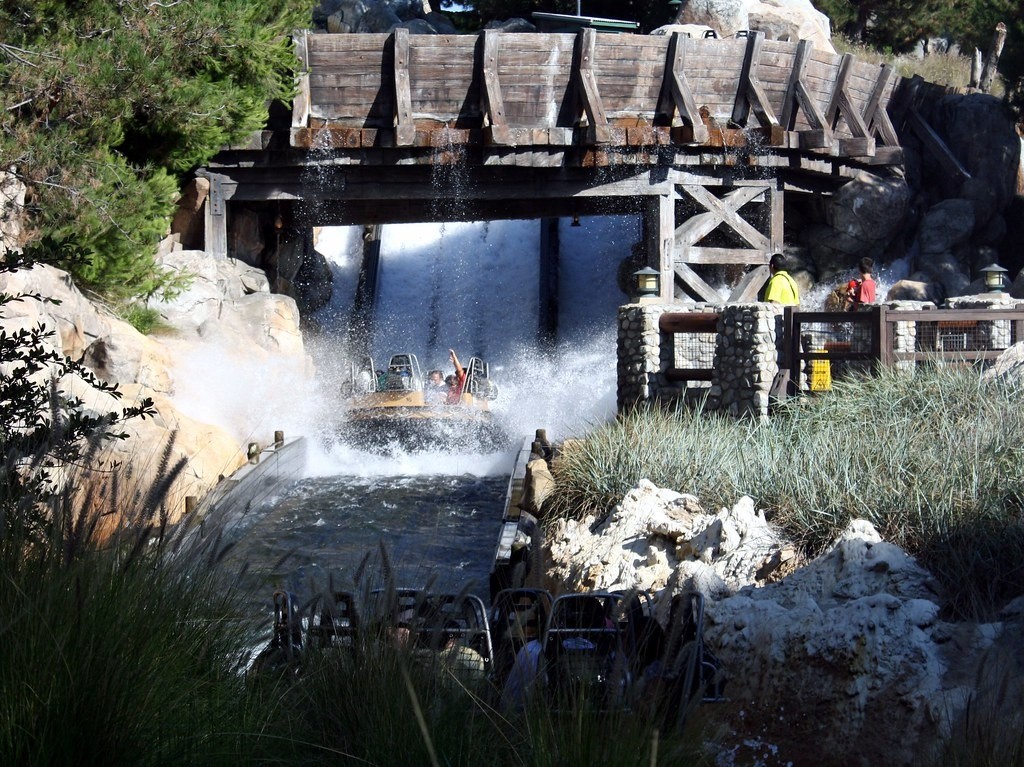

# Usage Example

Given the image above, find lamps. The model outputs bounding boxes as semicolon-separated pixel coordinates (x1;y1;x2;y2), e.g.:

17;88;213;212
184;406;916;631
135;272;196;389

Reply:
981;261;1008;294
635;267;661;299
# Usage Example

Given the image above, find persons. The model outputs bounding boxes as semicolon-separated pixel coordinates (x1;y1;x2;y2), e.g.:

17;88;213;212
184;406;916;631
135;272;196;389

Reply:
361;349;467;405
848;257;876;312
763;253;800;306
326;598;665;723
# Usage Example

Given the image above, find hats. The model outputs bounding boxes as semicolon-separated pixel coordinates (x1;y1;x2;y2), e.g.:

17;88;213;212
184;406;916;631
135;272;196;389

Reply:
846;280;861;290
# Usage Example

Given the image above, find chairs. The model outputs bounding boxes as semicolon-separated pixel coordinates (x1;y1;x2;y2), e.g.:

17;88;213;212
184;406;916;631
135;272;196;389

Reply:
271;586;731;745
350;354;493;398
